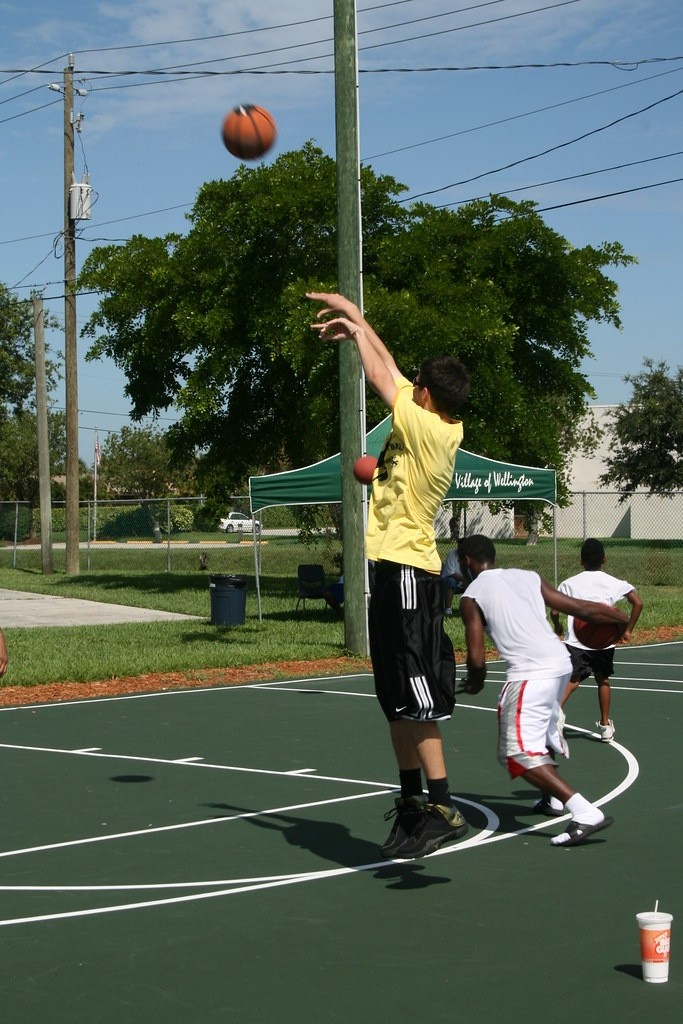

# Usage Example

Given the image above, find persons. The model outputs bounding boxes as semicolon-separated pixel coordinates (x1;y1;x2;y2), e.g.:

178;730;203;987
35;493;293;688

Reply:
551;538;643;742
455;535;614;846
306;293;472;858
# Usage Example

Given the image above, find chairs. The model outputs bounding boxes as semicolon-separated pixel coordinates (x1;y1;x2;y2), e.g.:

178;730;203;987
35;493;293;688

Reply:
295;564;329;615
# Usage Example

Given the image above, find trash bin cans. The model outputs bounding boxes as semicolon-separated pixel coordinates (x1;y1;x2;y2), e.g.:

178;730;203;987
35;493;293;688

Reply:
207;573;248;625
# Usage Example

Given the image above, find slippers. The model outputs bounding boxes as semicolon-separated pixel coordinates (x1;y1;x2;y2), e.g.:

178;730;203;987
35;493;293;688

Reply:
550;817;613;846
531;799;565;816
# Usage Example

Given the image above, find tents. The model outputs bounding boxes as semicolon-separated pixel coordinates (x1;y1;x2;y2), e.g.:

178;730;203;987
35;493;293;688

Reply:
250;411;557;622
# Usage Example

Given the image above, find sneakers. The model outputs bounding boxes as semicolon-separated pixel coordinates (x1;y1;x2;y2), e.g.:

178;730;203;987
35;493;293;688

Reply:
595;719;615;742
396;803;468;858
380;795;428;857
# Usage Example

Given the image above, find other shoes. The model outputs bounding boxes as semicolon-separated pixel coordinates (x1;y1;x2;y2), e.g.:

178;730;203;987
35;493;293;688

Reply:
446;608;452;616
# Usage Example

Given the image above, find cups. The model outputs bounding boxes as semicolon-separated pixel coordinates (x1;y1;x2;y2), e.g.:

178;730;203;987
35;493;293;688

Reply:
636;911;673;983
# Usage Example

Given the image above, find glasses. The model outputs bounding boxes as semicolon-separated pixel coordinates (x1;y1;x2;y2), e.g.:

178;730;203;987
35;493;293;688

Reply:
413;370;432;391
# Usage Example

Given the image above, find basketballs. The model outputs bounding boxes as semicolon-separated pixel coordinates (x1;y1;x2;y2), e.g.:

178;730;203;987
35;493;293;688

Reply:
353;455;378;485
220;103;278;160
572;603;625;652
